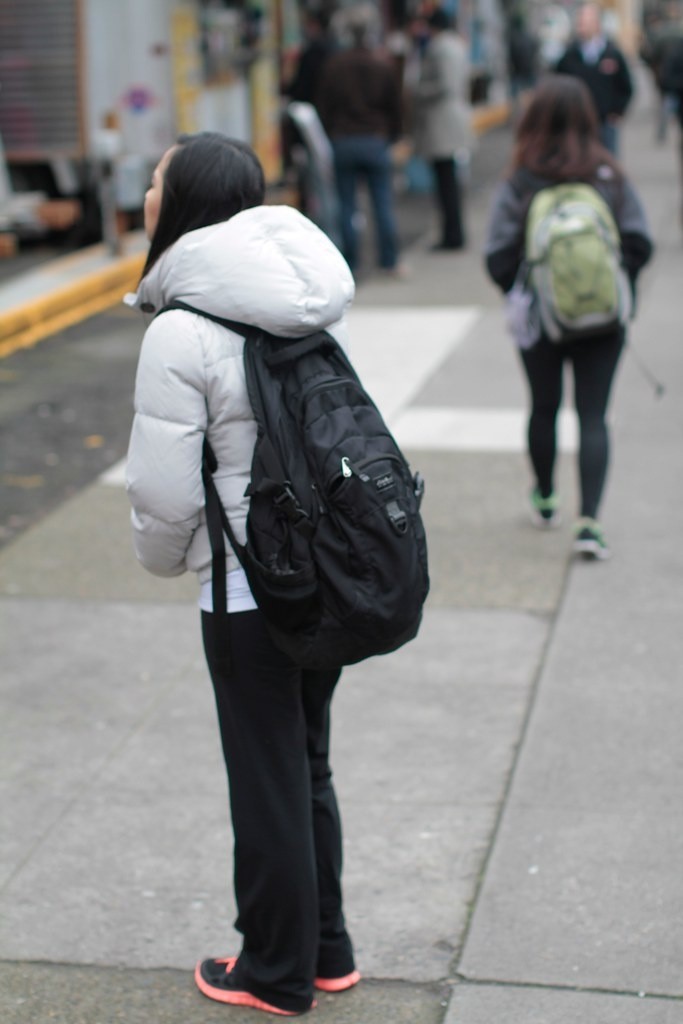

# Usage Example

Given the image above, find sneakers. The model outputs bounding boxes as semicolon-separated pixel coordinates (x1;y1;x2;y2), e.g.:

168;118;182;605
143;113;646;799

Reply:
575;515;612;561
195;957;361;1016
532;485;556;526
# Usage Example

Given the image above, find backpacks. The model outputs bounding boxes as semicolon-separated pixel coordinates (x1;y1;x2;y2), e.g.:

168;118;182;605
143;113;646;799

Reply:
153;302;430;671
513;158;631;346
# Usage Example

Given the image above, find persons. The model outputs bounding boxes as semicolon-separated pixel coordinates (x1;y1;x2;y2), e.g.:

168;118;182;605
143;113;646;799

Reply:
278;0;683;277
484;77;653;560
124;132;363;1016
